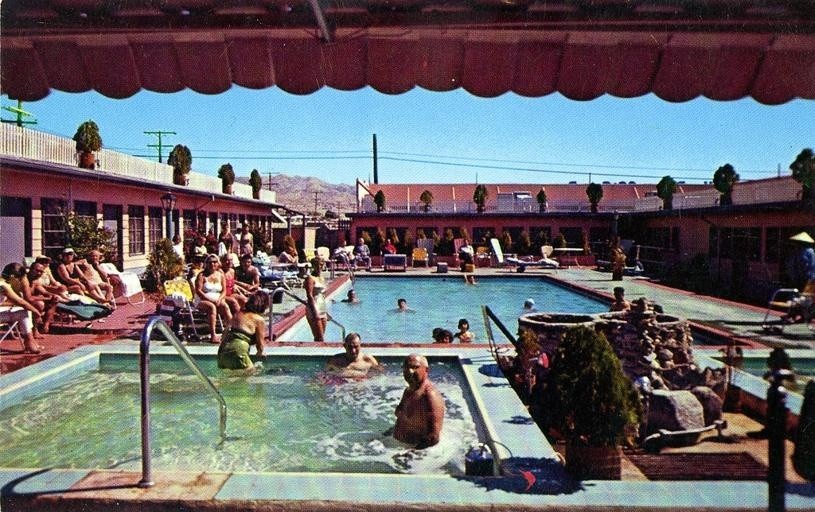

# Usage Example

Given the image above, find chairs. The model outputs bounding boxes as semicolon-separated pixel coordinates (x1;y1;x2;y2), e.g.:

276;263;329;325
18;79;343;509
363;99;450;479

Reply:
411;239;560;275
761;278;815;334
1;256;146;356
163;243;370;341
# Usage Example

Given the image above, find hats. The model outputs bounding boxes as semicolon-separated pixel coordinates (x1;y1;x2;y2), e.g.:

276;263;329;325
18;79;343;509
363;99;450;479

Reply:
36;254;52;264
63;248;74;253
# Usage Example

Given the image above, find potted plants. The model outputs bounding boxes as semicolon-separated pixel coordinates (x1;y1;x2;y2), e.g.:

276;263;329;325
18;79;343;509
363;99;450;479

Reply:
167;143;263;200
71;119;103;170
527;326;643;479
656;147;815;208
373;182;603;213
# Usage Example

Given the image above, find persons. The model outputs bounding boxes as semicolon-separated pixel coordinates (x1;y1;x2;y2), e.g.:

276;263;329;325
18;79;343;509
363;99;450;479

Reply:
331;239;349;270
389;298;414;314
467;275;477;285
215;289;270;370
381;352;444;450
430;327;442;344
453;317;480;343
278;246;298;263
172;222;271;344
324;331;387;378
380;239;396;272
456;239;474;263
352;237;372;272
331;289;361;305
0;249;114;353
788;232;815;281
608;286;630;311
436;330;453;344
305;257;327;342
524;297;538;313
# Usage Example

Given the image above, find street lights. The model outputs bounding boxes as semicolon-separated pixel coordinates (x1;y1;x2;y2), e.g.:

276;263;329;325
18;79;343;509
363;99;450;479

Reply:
160;192;178;240
284;211;292;235
613;209;618;236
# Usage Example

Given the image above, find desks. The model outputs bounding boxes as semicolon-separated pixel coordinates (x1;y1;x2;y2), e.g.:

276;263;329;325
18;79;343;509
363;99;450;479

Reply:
554;247;584;269
